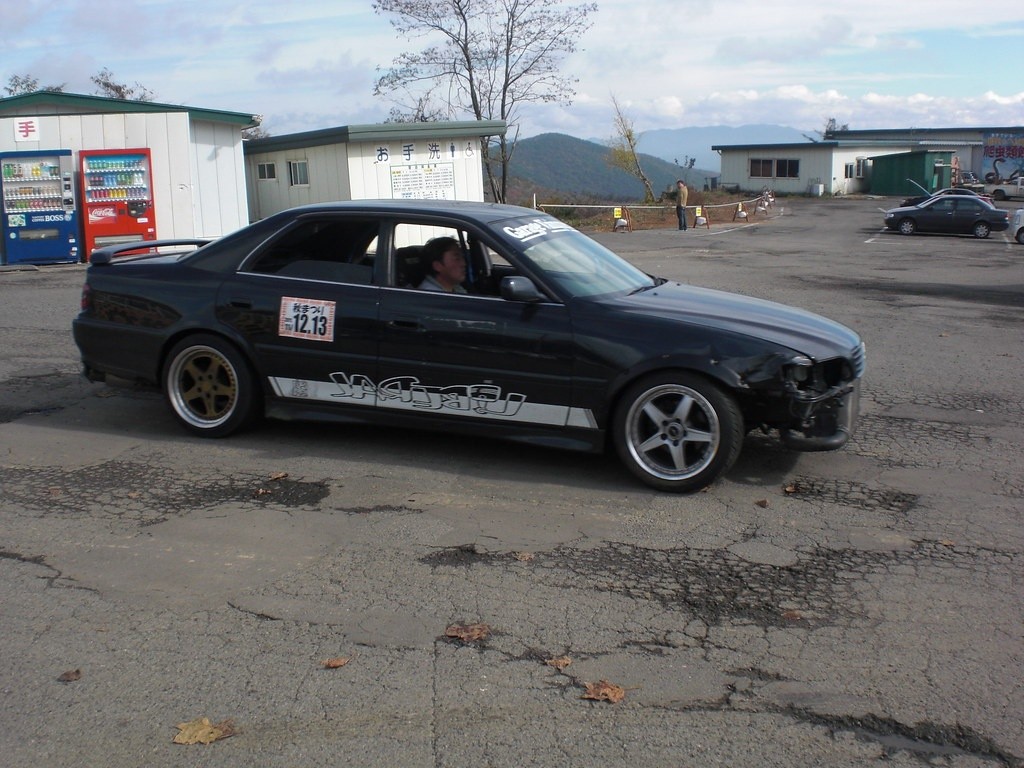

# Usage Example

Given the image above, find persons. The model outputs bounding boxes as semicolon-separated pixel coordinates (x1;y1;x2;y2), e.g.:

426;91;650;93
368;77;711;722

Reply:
674;180;688;231
409;238;471;296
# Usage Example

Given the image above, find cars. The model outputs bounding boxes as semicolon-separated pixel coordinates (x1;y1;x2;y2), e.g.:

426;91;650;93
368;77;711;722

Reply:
69;200;867;496
880;176;1024;244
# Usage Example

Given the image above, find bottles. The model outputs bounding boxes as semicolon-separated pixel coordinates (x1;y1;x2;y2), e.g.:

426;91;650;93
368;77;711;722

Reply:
3;162;61;208
88;159;146;199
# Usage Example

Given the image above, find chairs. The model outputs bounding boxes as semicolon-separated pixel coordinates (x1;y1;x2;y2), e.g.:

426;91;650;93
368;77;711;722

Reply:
394;246;428;289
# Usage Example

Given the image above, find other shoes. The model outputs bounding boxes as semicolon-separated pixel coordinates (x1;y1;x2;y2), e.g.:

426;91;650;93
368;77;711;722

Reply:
677;228;687;232
